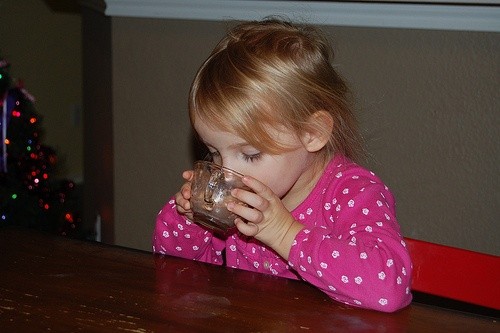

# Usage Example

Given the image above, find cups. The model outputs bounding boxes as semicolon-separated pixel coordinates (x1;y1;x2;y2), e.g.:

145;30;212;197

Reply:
189;161;256;237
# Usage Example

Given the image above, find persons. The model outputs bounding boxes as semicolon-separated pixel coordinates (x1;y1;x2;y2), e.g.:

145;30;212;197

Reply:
151;19;415;313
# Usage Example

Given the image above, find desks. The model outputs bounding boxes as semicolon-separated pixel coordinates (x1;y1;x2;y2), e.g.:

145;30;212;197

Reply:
1;220;500;333
